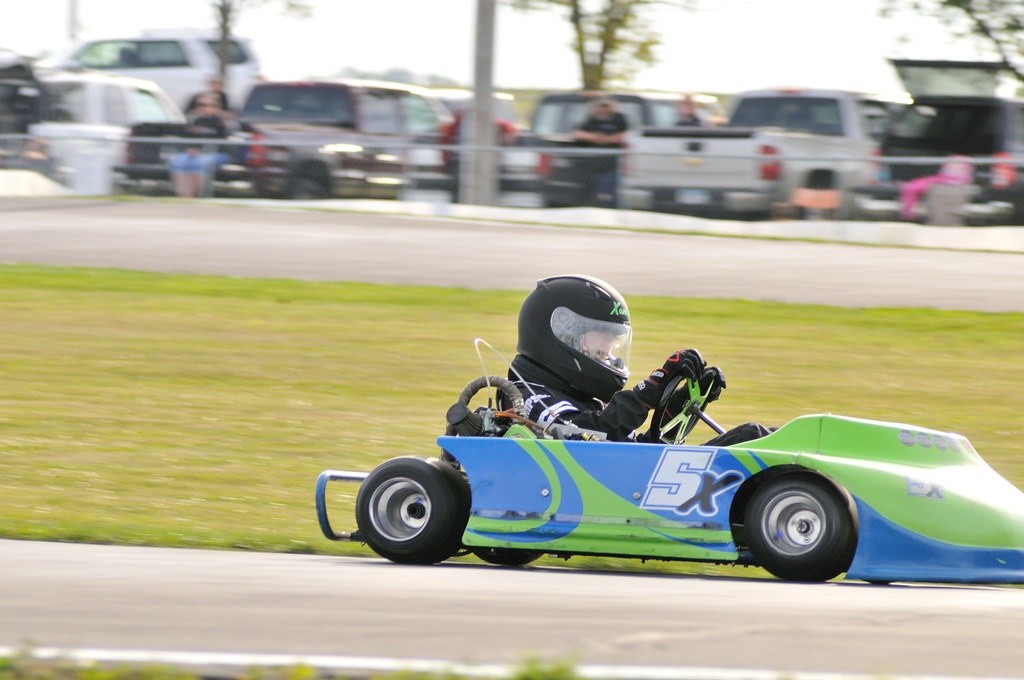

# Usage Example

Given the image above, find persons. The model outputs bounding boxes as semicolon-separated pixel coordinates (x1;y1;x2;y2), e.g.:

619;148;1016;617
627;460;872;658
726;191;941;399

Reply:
509;274;781;445
676;103;700;125
187;73;237;131
573;101;627;205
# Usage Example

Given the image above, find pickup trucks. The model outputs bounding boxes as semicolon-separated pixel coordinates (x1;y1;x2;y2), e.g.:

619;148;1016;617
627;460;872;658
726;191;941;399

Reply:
109;79;456;199
615;90;893;212
400;92;726;207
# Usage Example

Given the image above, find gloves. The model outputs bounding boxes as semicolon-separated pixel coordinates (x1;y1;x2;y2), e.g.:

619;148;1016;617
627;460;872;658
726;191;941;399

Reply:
662;347;707;381
698;366;726;403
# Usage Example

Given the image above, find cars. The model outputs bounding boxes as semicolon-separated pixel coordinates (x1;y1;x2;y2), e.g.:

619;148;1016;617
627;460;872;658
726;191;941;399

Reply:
881;59;1024;224
30;34;267;112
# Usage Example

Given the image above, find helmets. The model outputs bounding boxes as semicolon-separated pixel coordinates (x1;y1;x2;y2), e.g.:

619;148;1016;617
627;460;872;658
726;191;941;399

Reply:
516;274;633;405
